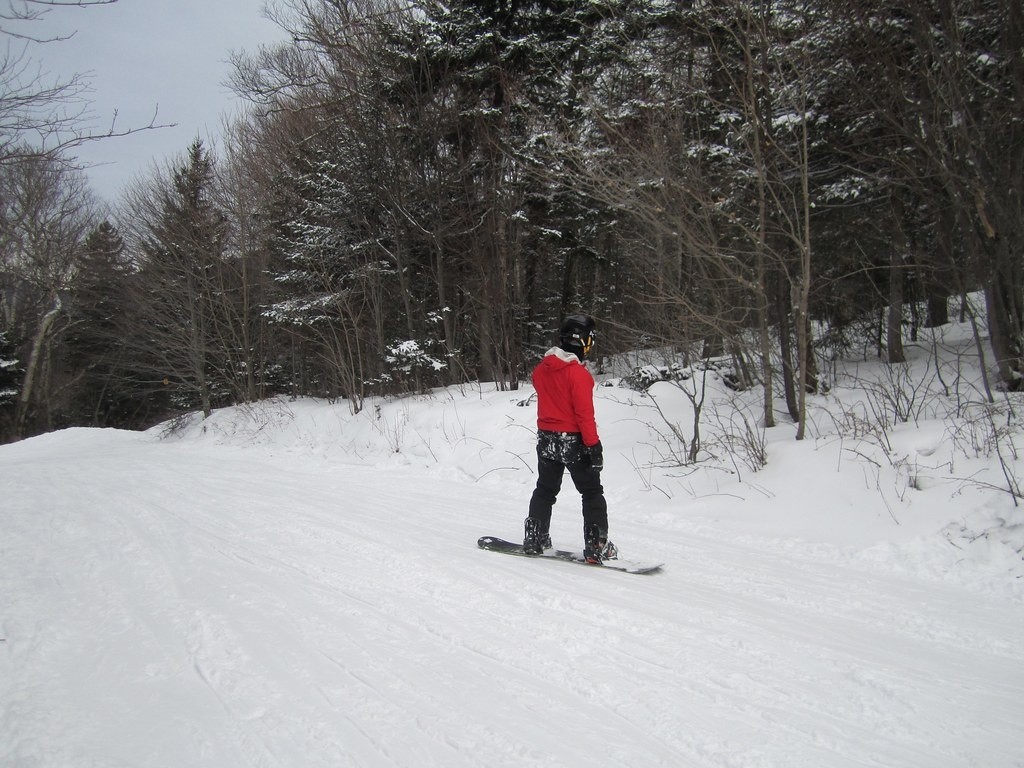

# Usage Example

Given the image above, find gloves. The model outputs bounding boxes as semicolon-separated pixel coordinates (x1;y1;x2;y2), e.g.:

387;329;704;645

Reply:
586;440;603;472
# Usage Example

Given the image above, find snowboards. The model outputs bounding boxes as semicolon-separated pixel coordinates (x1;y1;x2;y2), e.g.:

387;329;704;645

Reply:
476;534;667;577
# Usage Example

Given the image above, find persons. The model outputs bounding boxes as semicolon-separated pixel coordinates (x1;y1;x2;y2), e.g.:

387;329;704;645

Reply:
523;314;616;562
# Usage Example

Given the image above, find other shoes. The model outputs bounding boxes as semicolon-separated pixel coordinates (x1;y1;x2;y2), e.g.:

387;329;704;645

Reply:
523;533;552;554
583;538;617;563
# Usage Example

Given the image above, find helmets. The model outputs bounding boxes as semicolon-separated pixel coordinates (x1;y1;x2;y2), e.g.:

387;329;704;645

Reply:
558;315;597;340
